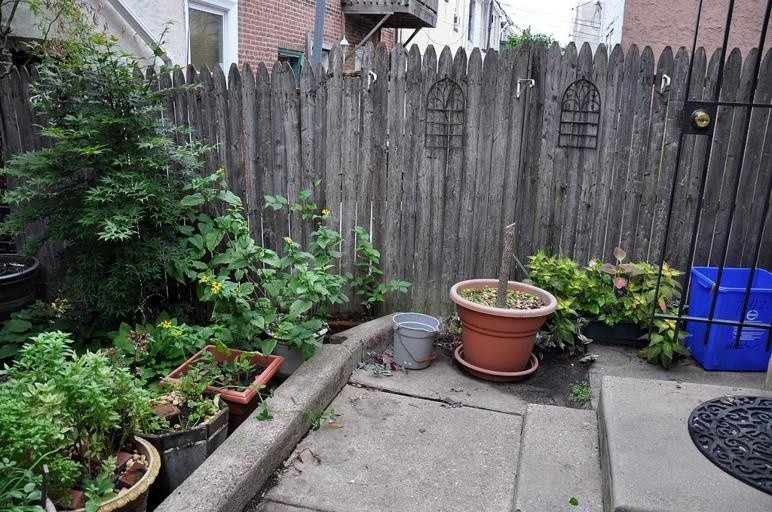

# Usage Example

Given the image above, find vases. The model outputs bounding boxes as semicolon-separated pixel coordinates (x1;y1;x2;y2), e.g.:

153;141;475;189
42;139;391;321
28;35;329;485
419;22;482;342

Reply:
266;312;328;376
0;254;40;318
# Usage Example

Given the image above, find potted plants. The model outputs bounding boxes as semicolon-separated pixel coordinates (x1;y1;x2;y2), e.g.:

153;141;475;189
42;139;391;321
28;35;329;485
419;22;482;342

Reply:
159;339;284;433
0;330;165;512
450;222;558;373
527;247;691;371
132;374;230;490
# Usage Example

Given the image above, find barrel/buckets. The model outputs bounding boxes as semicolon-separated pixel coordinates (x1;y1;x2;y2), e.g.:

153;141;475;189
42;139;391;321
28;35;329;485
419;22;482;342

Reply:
392;312;440;370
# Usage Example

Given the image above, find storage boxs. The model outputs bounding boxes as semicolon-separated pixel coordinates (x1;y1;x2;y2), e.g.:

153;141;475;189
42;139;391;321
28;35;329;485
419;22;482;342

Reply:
685;266;772;371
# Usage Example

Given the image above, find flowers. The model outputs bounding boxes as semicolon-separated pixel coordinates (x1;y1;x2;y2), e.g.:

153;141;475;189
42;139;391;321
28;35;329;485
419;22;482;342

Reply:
179;167;350;361
